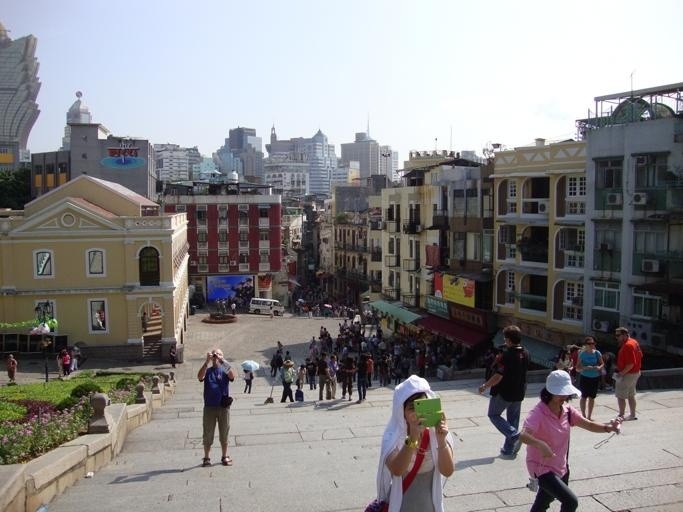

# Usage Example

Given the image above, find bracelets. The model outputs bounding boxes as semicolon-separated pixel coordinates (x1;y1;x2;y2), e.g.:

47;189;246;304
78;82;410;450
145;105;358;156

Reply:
481;383;486;390
437;445;449;452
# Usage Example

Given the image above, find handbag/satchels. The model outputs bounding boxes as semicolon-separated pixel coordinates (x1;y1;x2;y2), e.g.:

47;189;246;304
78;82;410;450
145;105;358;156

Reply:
363;498;390;512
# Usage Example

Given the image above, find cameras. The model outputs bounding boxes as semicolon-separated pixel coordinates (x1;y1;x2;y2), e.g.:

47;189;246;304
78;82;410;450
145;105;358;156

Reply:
526;477;538;492
616;424;621;434
211;353;219;357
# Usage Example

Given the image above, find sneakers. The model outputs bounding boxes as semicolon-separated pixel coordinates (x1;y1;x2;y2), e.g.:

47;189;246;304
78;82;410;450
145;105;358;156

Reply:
499;447;516;459
506;431;522;444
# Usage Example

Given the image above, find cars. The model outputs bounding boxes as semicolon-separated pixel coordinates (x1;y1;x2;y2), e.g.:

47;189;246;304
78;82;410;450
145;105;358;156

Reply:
246;297;286;317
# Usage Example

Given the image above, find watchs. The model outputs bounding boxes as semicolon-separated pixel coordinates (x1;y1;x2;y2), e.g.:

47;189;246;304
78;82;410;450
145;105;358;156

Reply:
404;435;419;449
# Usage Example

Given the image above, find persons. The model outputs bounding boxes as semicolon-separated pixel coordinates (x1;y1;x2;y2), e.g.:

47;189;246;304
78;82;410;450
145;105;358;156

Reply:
375;376;454;512
612;327;643;420
71;347;80;370
554;345;614;392
140;312;147;332
574;337;604;421
477;326;530;457
167;346;176;368
213;278;465;406
5;355;17;382
197;350;233;469
63;351;70;375
517;369;621;511
56;354;63;379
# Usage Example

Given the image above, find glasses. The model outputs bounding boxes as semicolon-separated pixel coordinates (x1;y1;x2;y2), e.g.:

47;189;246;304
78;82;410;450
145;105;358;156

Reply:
615;334;624;337
585;343;596;346
502;335;511;339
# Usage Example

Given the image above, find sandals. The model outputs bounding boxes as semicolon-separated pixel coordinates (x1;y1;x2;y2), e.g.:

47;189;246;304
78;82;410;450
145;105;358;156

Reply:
221;455;233;465
203;457;212;467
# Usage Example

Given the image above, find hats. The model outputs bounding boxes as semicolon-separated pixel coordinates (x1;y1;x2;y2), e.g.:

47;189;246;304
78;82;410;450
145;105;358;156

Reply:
545;369;584;397
282;360;295;366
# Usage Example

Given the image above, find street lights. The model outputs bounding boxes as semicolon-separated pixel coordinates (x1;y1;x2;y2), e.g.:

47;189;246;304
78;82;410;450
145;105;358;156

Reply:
34;299;54;385
382;152;392;188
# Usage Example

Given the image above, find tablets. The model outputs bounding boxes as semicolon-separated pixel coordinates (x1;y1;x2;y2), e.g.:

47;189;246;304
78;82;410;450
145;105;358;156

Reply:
414;398;443;427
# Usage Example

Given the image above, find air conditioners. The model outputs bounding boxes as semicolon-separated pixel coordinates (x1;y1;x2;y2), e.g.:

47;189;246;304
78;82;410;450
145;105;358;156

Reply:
188;261;198;266
537;199;550;214
606;192;623;206
645;331;668;351
415;225;424;233
641;258;661;273
631;191;648;205
229;260;236;266
376;222;385;230
591;318;613;333
